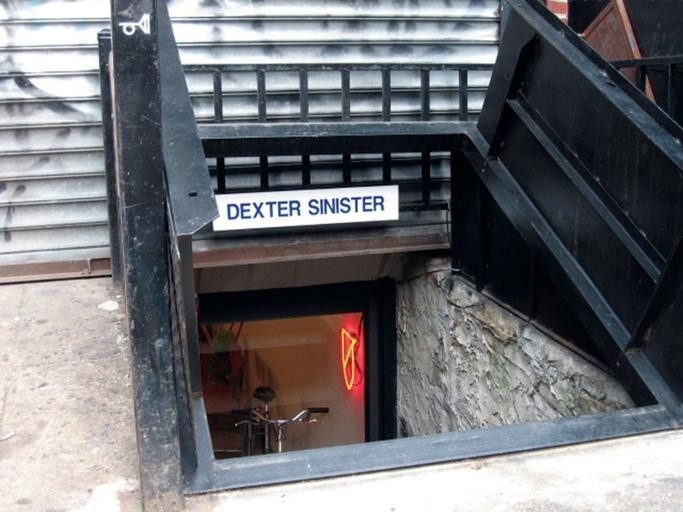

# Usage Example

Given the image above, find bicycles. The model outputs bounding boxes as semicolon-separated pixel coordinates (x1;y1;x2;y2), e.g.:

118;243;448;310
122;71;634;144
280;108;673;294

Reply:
220;386;329;454
